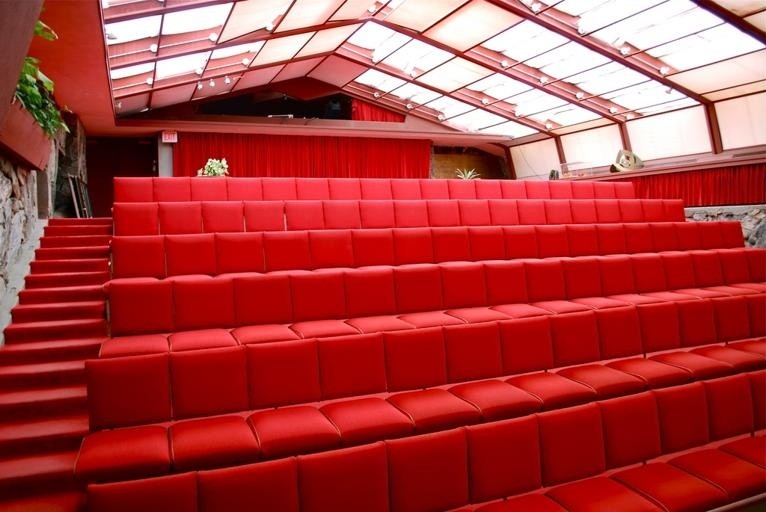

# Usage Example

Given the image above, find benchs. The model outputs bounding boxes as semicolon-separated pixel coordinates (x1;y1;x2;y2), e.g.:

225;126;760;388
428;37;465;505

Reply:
73;176;766;512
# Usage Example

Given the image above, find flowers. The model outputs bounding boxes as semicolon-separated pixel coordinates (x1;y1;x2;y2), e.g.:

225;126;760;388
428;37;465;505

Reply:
197;157;229;177
